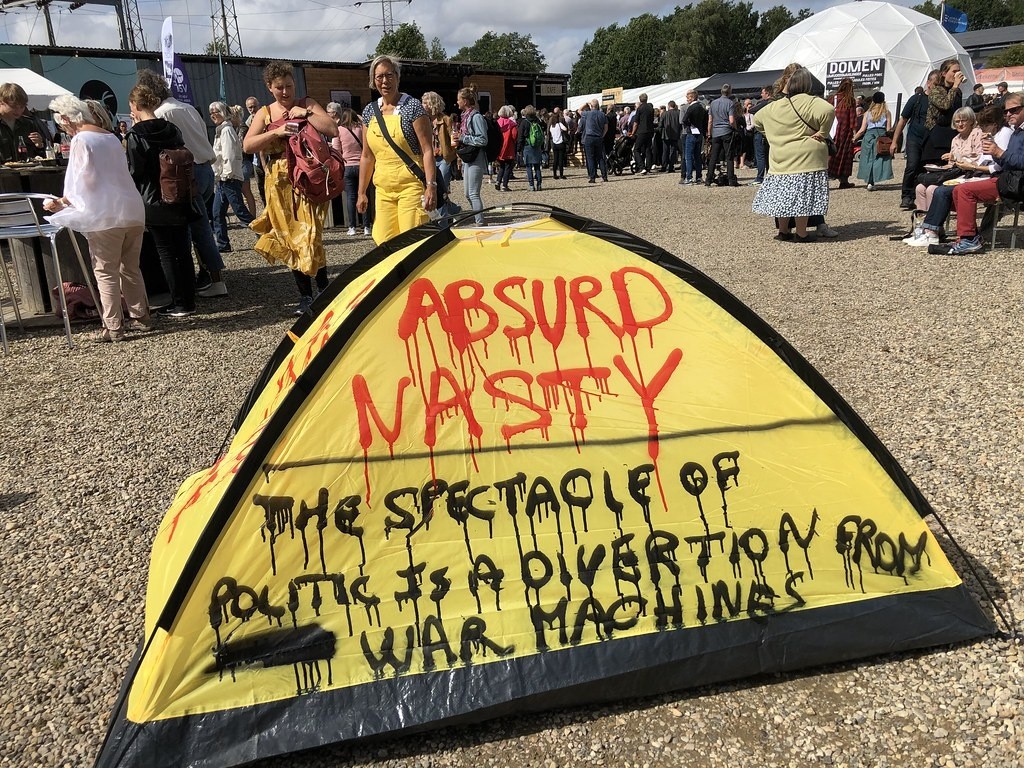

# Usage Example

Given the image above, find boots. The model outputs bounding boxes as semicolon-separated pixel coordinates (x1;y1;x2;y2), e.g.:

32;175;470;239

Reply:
554;169;567;179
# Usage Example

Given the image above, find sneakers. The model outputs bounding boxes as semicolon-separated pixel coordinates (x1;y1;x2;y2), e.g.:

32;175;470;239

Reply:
640;164;662;174
815;224;838;237
953;235;984;255
900;197;915;208
346;227;372;236
866;184;877;191
903;230;940;246
747;180;762;187
294;288;323;318
156;302;197;317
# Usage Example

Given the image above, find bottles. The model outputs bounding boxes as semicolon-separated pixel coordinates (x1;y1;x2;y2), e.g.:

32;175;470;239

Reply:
451;129;458;146
60;133;70;159
18;136;27;163
421;195;442;220
45;138;55;159
66;135;72;145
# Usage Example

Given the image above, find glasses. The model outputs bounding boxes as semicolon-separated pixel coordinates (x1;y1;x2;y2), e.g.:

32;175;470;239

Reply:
1003;105;1024;114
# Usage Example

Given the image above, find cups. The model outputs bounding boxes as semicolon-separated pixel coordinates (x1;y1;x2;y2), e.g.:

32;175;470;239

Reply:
982;138;994;155
954;71;967;83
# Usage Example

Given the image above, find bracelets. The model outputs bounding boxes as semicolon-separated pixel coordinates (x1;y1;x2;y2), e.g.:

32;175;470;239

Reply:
425;181;437;187
994;148;1001;156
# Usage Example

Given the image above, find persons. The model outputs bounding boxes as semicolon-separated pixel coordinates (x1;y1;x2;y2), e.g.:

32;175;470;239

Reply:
243;62;340;315
210;86;488;254
356;55;438;246
890;60;1024;255
486;64;908;190
0;69;228;344
752;69;836;243
751;64;839;243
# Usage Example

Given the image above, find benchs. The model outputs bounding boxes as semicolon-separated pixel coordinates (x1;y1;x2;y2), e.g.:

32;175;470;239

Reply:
944;198;1022;250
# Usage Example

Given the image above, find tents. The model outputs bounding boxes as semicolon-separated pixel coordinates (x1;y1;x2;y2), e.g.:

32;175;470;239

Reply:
95;204;1008;768
746;2;980;154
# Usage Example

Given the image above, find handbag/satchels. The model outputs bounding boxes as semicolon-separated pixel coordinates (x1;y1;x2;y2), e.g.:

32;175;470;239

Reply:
456;139;480;164
877;135;893;155
829;95;839;140
425;165;449;210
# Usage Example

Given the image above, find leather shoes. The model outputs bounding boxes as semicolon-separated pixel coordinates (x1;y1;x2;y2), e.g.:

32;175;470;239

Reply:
196;282;228;298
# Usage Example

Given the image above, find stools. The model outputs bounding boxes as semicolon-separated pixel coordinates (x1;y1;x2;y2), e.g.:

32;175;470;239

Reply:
0;192;107;356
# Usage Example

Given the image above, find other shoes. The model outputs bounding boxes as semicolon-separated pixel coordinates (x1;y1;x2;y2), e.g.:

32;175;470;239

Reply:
529;185;541;191
839;182;855;189
774;233;817;242
495;181;512;192
678;178;741;186
589;177;608;183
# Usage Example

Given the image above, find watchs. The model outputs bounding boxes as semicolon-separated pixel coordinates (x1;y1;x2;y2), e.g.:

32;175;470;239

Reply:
972;164;977;173
304;110;313;118
60;198;68;209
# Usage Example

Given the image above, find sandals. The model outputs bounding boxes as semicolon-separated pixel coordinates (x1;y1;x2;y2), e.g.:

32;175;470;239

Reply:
89;316;154;342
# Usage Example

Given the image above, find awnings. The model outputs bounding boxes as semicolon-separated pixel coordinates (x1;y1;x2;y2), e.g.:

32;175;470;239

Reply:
567;77;709;111
0;68;73;97
693;68;824;101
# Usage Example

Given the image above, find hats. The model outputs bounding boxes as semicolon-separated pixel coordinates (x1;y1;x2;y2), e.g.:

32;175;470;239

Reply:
995;81;1008;88
872;92;884;103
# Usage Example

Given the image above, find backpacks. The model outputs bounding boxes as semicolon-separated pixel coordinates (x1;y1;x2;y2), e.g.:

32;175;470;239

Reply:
263;96;347;221
525;119;543;148
159;147;195;204
470;112;504;162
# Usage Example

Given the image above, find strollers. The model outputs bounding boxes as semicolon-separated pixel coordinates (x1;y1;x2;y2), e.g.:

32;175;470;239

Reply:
605;133;638;176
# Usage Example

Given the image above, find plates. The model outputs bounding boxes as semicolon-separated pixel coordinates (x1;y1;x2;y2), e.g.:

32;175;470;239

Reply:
924;166;947;171
943;179;965;186
